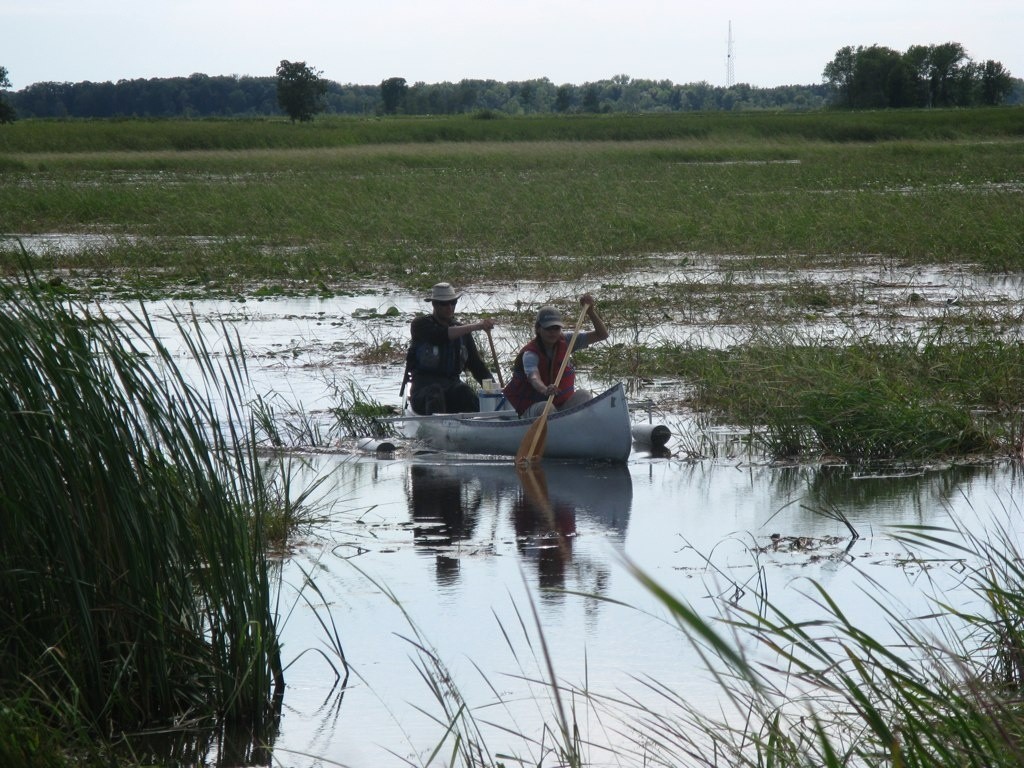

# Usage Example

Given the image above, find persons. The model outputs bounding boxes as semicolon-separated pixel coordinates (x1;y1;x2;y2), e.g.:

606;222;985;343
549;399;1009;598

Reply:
406;281;500;416
502;294;609;416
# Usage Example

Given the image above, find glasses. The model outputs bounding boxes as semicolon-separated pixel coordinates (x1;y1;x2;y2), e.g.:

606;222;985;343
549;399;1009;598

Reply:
436;300;456;307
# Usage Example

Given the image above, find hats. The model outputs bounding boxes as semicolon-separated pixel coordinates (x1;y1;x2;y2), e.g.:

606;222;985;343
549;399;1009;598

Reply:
424;282;463;302
536;306;565;328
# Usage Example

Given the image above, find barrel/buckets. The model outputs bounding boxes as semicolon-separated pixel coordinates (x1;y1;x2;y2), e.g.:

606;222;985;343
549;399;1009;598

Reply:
478;393;503;411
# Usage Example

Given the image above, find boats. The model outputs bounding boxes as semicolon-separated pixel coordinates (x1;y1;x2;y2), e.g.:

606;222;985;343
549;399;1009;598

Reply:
394;384;633;462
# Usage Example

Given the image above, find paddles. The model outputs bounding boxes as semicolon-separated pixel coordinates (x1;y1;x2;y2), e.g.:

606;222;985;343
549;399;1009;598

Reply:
516;304;590;461
482;328;505;388
